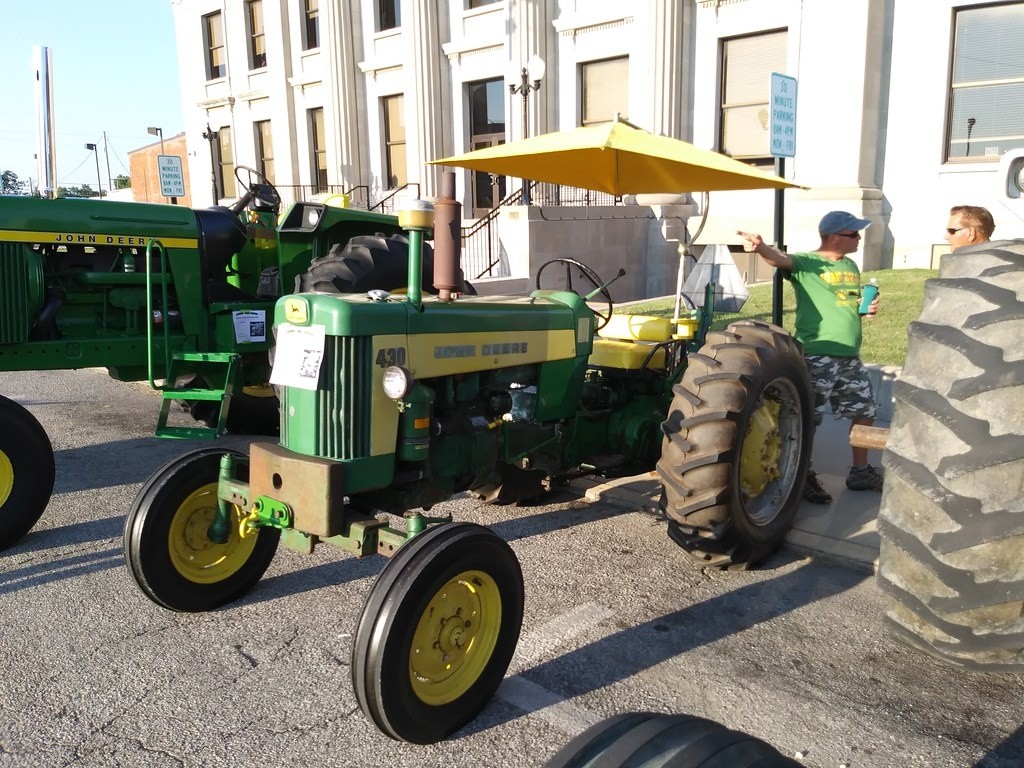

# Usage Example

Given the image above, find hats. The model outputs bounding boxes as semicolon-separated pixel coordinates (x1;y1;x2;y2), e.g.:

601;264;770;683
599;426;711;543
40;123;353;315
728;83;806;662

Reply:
819;211;872;236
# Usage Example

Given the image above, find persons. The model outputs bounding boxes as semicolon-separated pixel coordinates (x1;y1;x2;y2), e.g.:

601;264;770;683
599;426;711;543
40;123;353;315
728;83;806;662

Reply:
944;205;996;253
735;211;883;505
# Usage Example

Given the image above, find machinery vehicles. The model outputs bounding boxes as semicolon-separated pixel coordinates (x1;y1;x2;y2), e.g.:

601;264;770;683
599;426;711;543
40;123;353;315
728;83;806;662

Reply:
119;115;826;746
0;163;477;557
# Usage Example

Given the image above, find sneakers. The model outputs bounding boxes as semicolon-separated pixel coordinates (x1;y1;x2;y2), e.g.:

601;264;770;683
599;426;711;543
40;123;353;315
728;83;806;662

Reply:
802;469;833;504
846;464;884;490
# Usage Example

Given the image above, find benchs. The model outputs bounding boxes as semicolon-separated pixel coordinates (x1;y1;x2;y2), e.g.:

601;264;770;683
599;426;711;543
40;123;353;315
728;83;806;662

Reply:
581;312;671;373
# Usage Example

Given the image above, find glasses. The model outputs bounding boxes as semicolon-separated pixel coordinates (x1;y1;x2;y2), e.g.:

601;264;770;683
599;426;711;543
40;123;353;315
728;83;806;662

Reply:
946;227;967;234
837;232;859;238
